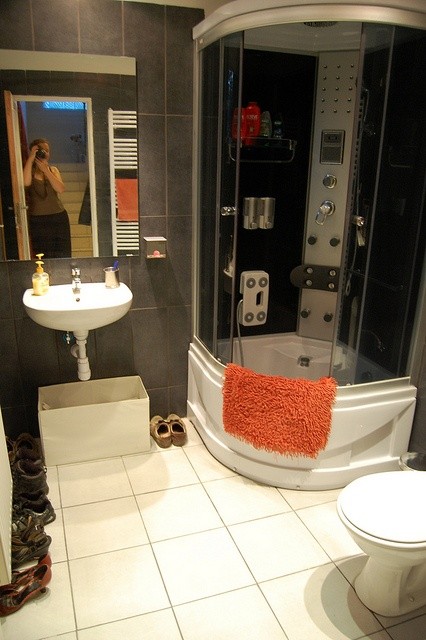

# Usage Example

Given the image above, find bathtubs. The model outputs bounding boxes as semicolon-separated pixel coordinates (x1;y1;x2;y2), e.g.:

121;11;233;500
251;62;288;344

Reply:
184;331;418;493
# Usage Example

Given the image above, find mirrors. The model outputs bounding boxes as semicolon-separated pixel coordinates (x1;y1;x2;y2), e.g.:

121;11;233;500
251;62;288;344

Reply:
0;94;98;261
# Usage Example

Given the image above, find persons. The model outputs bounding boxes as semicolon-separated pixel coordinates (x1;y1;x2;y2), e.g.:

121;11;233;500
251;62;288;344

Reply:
23;137;72;260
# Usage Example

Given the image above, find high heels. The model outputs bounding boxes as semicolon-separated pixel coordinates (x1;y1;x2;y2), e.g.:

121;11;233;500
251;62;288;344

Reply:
11;523;52;568
0;563;52;617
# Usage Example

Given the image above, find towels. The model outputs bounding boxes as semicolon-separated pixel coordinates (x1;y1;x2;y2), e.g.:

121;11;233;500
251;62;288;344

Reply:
222;363;335;458
114;177;138;224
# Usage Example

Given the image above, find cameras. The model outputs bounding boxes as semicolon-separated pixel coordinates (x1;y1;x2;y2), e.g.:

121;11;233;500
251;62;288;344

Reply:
35;145;46;161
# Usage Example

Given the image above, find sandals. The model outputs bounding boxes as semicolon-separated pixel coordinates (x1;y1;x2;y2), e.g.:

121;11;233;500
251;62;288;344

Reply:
11;555;51;582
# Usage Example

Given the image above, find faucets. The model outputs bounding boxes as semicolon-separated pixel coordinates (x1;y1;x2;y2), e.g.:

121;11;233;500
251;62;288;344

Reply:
71;267;81;284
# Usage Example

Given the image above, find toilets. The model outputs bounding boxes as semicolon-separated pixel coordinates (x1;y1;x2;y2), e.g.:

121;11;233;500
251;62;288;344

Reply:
335;468;425;618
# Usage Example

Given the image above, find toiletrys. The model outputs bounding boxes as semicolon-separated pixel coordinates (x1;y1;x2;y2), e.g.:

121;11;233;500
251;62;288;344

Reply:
31;260;50;296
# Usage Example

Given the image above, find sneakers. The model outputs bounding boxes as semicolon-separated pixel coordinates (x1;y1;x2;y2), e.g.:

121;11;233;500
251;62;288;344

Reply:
6;432;41;465
166;413;187;447
12;489;56;535
150;414;171;448
11;460;49;495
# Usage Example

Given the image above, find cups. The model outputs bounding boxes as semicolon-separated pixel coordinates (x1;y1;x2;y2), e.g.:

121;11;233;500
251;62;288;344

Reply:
103;267;120;288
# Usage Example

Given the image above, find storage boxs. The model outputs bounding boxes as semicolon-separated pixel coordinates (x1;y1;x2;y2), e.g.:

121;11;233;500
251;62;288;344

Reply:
36;373;151;468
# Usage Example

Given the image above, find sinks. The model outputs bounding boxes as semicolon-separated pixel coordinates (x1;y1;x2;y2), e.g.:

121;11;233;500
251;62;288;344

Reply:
22;281;134;332
